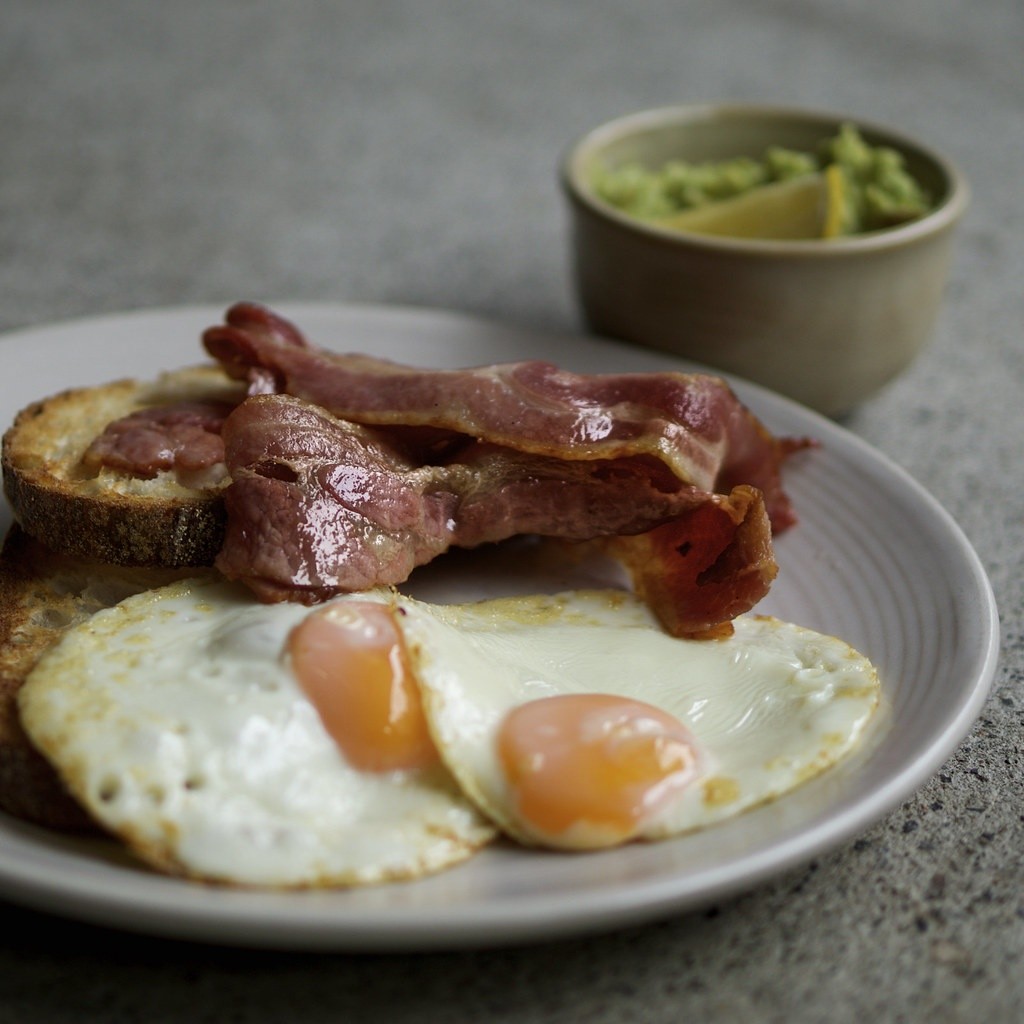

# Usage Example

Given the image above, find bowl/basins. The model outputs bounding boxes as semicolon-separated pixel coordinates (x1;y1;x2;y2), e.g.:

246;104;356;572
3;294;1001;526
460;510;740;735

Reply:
560;105;970;416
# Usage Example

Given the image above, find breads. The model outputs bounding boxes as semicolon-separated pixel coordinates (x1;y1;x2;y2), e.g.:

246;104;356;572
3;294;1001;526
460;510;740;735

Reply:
0;518;184;835
0;362;272;572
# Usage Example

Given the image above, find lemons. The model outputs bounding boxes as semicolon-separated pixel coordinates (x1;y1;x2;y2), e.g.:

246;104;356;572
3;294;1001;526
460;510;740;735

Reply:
647;162;858;240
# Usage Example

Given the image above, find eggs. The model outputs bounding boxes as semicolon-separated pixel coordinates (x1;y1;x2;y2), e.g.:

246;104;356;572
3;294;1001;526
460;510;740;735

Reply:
18;579;504;886
388;584;882;853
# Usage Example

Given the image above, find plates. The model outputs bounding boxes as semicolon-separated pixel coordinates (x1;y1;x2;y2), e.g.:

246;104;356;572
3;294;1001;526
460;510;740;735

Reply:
0;299;999;946
